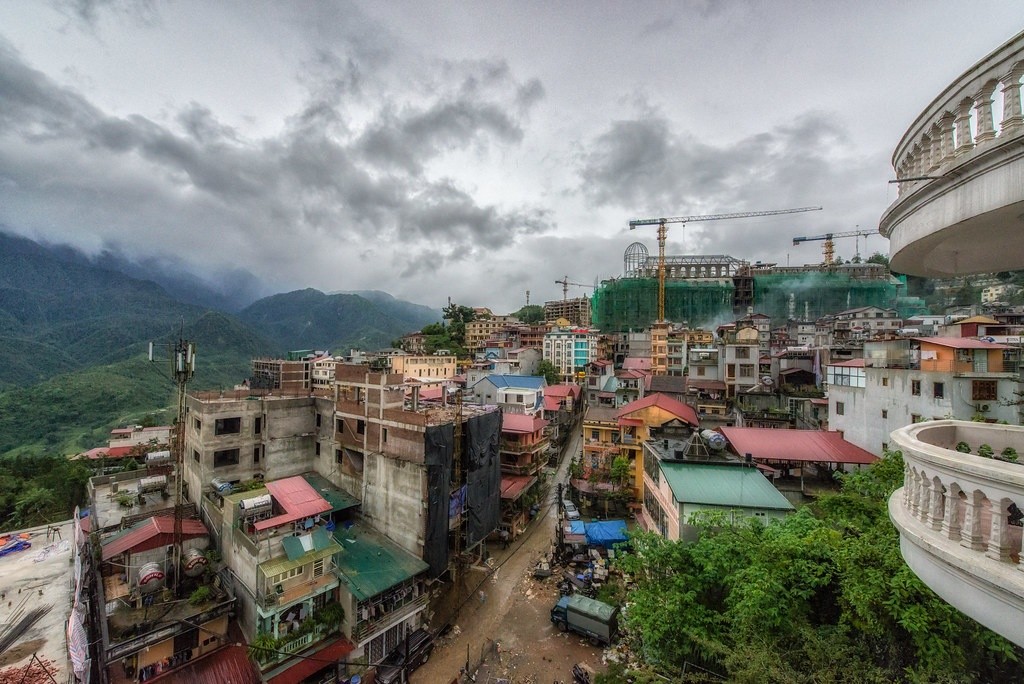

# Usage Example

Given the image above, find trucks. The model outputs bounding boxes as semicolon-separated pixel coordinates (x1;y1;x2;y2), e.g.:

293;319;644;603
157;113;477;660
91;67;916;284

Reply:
548;593;621;647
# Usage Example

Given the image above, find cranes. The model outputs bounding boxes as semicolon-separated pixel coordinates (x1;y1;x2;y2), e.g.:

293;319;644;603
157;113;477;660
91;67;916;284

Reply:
627;203;824;322
554;274;596;318
789;227;881;267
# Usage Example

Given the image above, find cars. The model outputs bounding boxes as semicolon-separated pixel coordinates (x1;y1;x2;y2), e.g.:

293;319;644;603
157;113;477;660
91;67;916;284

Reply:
561;498;581;521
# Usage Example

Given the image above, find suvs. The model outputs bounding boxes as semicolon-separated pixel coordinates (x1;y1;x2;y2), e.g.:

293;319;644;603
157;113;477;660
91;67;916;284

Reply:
374;626;437;684
